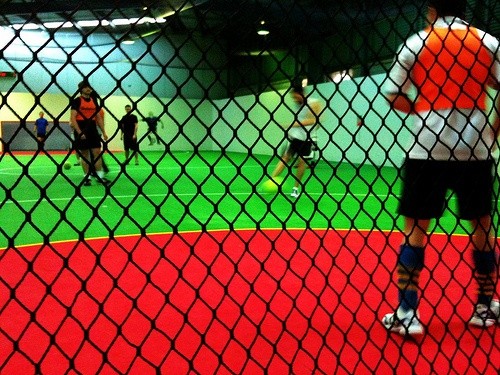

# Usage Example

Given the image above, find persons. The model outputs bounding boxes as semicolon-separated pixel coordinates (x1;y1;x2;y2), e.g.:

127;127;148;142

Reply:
272;85;320;197
120;105;139;165
34;111;49;142
382;0;500;334
69;80;113;186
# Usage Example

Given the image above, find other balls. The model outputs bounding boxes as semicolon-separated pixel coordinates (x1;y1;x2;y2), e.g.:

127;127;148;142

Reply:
64;162;71;169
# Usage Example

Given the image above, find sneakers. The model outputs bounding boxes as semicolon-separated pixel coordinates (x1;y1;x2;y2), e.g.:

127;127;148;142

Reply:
469;300;500;327
135;160;138;164
84;179;90;186
97;179;109;184
291;187;297;197
382;307;422;334
73;163;80;166
